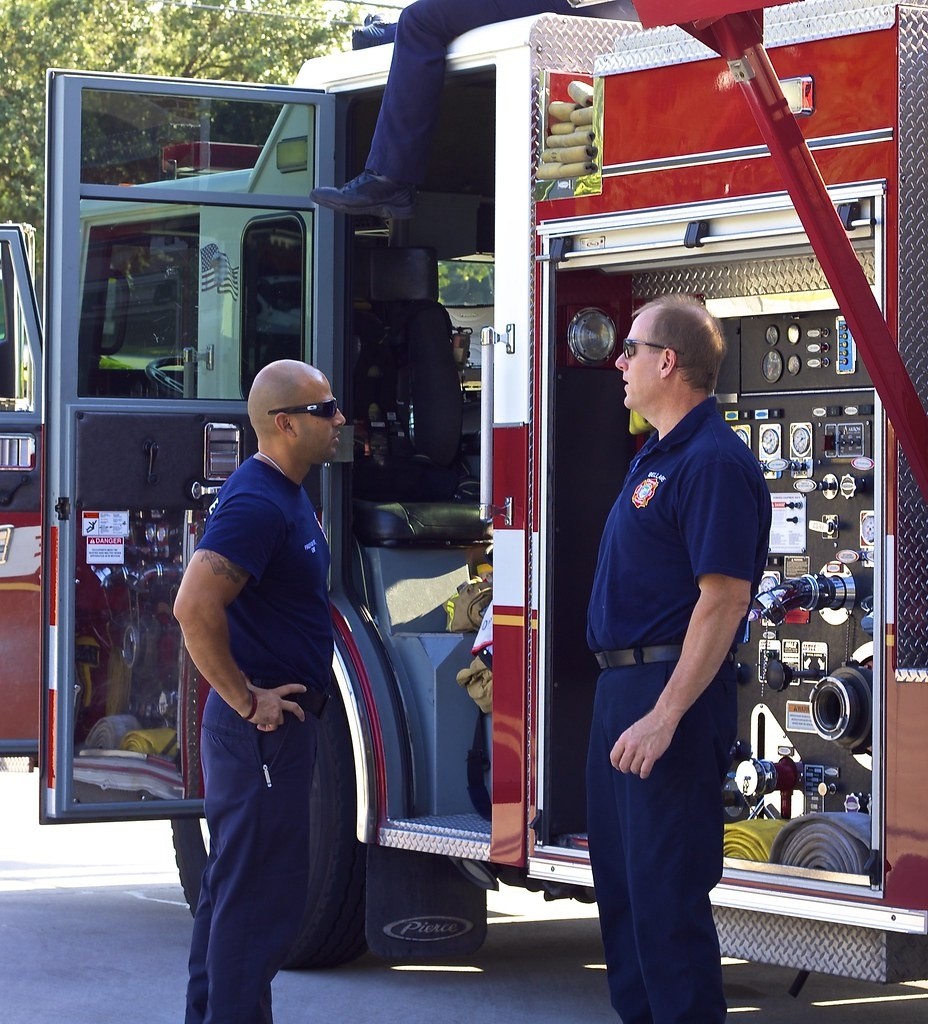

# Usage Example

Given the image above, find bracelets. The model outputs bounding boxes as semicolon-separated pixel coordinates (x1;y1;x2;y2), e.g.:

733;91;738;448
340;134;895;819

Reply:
243;689;258;720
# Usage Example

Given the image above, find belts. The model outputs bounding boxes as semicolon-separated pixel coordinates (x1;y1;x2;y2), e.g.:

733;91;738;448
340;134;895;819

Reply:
595;648;734;669
262;681;332;719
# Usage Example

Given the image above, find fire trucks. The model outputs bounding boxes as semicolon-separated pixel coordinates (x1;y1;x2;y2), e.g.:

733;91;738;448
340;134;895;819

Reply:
1;2;928;987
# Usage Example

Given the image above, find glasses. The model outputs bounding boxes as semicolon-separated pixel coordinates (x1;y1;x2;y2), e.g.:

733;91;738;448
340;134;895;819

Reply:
623;339;684;359
268;398;338;418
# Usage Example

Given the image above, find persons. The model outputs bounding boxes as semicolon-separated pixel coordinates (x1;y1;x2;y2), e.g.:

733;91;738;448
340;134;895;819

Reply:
309;0;580;221
585;295;773;1023
174;360;346;1024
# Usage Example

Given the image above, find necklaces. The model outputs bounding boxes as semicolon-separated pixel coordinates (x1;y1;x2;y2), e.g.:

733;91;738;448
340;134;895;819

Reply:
258;450;286;478
